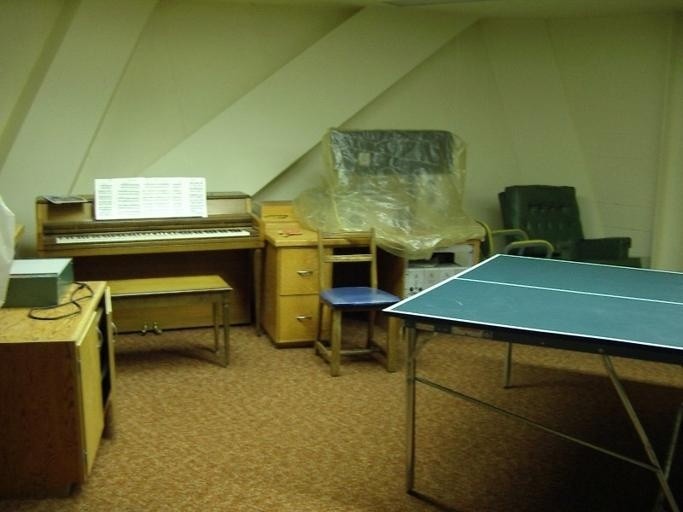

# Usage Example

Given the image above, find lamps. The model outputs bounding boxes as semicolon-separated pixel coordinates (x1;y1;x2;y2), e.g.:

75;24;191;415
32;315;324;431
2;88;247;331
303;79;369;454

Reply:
474;218;553;260
315;227;400;377
498;185;641;268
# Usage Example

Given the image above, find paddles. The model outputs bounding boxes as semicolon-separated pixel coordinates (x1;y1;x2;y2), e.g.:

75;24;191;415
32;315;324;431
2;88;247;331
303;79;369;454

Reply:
0;322;682;512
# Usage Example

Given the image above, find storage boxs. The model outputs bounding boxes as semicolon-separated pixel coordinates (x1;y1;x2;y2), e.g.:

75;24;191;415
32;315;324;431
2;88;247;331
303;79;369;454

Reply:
106;275;232;365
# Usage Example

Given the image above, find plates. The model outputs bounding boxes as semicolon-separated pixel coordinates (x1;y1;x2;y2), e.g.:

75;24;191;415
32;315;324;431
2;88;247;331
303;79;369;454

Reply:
0;282;118;498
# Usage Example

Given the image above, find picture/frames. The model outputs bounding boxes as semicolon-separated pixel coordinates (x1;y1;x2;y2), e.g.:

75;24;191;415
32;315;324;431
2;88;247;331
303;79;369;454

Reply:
260;200;486;348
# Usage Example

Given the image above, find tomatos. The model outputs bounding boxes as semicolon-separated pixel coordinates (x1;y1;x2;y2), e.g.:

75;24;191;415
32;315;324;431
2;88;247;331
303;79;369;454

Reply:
36;192;265;338
